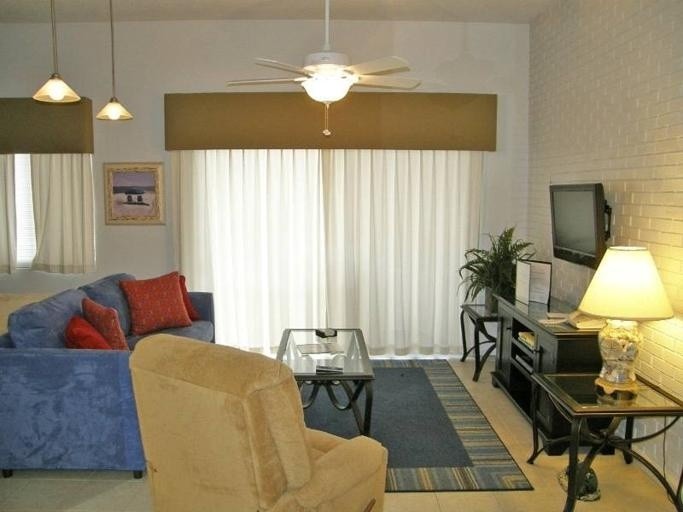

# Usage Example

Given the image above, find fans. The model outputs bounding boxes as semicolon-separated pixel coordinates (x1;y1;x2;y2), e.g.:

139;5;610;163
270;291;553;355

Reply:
226;1;420;94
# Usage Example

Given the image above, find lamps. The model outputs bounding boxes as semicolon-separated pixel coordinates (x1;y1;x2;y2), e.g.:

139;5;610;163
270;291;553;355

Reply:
31;1;82;103
95;0;133;121
303;74;355;136
578;246;675;398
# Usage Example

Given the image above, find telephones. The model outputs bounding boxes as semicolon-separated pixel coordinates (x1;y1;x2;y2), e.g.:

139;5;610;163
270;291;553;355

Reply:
567;310;607;329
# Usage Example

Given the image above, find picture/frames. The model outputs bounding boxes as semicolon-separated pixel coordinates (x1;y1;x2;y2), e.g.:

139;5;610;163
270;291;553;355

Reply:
102;161;165;226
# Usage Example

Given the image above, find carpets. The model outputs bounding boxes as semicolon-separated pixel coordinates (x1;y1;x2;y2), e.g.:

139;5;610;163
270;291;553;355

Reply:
282;359;534;492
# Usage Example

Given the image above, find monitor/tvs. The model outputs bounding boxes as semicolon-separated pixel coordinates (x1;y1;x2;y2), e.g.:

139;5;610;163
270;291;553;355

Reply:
548;184;611;270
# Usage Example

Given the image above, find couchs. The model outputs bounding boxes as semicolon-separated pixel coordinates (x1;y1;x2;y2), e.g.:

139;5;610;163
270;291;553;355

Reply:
127;334;388;512
0;272;214;479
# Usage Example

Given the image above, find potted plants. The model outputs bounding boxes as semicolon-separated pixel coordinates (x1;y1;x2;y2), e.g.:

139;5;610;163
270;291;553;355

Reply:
456;225;538;313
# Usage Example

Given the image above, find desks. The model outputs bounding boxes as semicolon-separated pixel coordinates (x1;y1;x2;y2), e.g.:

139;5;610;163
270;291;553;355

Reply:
528;371;683;512
460;304;500;381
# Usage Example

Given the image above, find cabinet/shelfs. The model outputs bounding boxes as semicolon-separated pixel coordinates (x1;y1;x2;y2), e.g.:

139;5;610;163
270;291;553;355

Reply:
489;294;619;456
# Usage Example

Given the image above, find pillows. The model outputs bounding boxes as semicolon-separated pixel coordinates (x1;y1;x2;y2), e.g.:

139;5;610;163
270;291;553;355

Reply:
180;275;203;321
7;289;86;349
64;316;111;351
117;271;192;336
82;296;130;350
80;274;136;337
